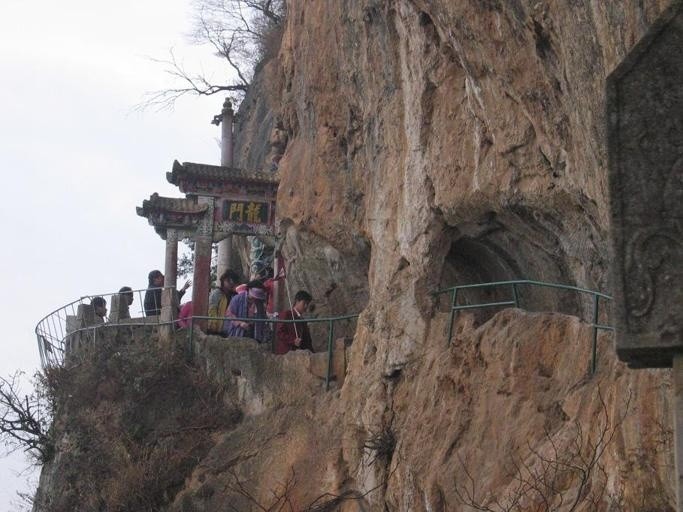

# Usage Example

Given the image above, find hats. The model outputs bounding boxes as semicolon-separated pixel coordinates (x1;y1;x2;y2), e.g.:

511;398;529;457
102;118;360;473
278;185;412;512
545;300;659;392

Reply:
248;287;266;301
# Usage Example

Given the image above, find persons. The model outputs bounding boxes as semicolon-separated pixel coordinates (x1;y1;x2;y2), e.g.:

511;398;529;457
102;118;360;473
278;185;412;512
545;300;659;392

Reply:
177;271;268;344
272;290;315;355
143;270;191;317
91;296;107;324
117;284;133;319
